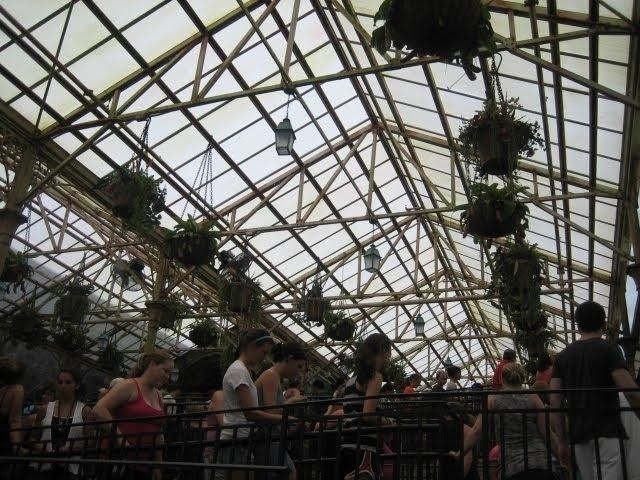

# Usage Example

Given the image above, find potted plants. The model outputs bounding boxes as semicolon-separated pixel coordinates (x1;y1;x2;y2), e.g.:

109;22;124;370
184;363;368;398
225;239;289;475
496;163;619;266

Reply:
322;312;358;342
96;342;124;369
113;258;146;290
162;215;222;268
1;249;35;293
451;93;555;372
48;279;94;326
294;282;332;325
185;321;223;348
95;165;168;220
49;316;90;350
218;275;265;318
9;295;48;347
143;298;189;331
369;0;497;80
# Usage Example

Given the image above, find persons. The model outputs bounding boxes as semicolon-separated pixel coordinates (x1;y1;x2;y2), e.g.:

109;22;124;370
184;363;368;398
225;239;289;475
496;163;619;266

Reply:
0;299;640;480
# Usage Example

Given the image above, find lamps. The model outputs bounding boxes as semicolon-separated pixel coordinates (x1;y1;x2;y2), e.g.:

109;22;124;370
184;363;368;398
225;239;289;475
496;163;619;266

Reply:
411;295;428;337
361;215;386;274
273;85;298;158
